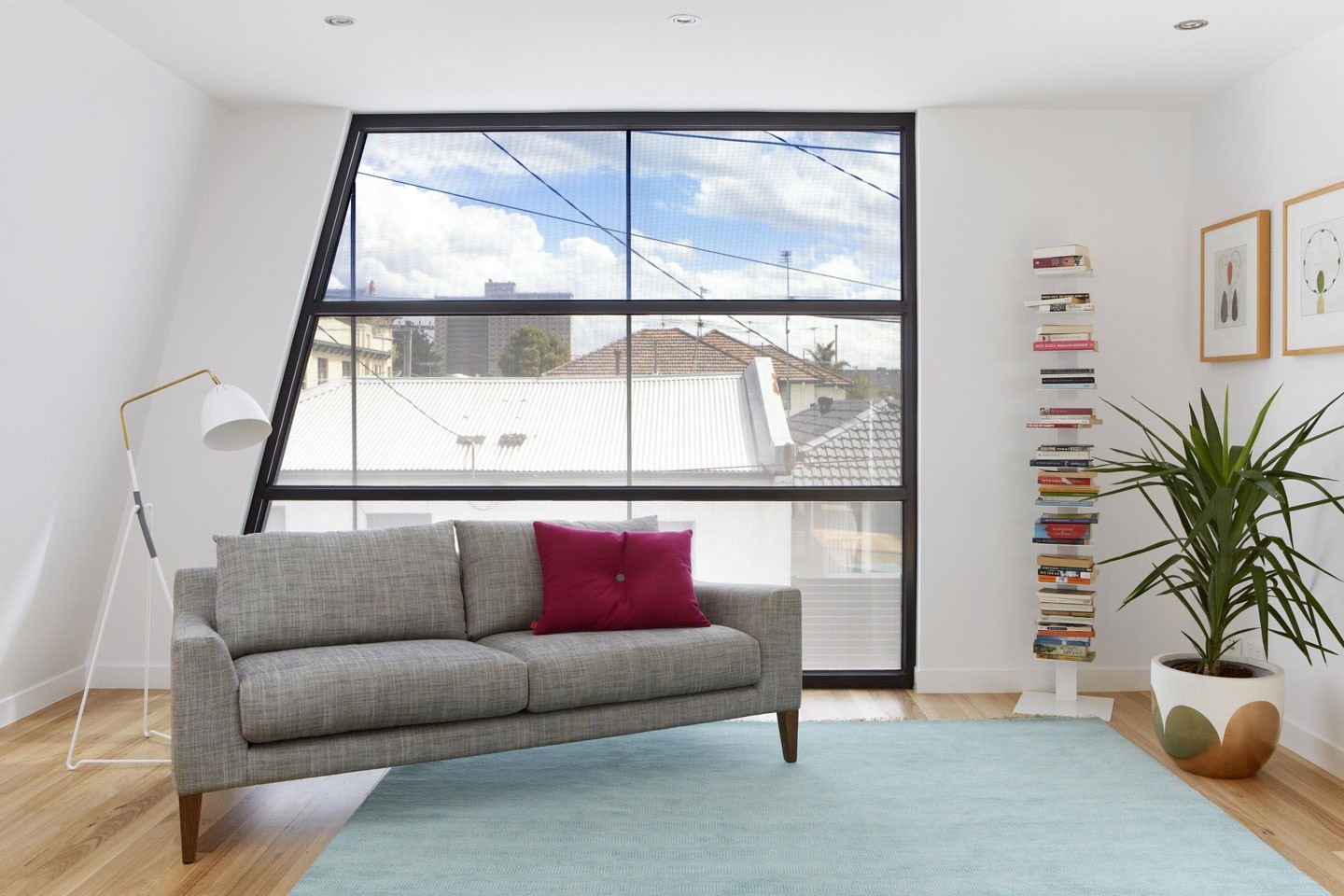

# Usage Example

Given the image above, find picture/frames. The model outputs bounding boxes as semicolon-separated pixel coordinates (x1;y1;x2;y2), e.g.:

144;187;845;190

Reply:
1282;182;1344;356
1199;210;1270;361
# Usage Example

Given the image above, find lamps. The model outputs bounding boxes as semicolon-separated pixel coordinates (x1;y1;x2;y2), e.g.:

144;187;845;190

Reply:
65;369;275;769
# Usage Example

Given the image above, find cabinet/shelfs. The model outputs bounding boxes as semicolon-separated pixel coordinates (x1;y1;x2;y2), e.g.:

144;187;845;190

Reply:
1014;271;1116;721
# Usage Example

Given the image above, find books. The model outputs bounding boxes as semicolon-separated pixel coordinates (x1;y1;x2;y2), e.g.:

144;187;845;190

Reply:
1025;242;1100;661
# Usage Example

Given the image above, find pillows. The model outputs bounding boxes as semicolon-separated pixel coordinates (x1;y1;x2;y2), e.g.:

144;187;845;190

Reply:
454;515;662;641
533;521;710;634
213;521;465;660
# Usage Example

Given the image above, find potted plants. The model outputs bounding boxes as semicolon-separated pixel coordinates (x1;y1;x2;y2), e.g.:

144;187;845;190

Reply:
1077;383;1343;779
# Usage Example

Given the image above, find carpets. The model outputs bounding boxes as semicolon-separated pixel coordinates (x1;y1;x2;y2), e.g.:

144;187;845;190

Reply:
286;717;1330;896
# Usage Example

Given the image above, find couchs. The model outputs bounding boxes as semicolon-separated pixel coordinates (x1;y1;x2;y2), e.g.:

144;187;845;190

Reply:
172;515;802;864
